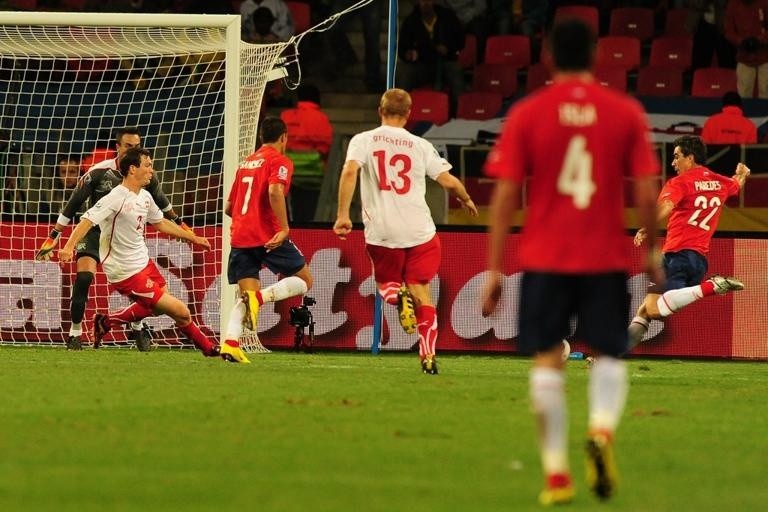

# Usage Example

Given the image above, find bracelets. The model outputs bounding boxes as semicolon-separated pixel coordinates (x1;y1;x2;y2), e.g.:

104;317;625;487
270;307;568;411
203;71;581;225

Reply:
456;195;470;204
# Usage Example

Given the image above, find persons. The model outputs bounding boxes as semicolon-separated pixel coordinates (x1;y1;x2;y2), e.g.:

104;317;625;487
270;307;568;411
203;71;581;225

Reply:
36;126;193;354
700;90;756;143
57;147;220;355
47;157;87;214
616;133;749;354
220;115;312;362
270;84;334;223
330;87;479;374
239;0;535;81
479;13;660;507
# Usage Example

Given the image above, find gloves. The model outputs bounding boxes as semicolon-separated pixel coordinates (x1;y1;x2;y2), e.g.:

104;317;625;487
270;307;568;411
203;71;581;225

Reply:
170;214;195;246
32;227;64;265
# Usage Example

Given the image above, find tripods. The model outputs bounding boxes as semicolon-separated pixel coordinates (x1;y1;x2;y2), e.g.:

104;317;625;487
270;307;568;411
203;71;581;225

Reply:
288;326;314;355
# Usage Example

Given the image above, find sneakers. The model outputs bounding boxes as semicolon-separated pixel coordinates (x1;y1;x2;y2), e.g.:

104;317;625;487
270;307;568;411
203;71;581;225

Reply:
705;271;746;298
583;431;619;500
239;289;260;332
219;342;252;365
202;345;222;358
395;286;417;337
92;312;111;350
536;485;577;506
131;321;153;353
420;353;438;375
66;334;82;351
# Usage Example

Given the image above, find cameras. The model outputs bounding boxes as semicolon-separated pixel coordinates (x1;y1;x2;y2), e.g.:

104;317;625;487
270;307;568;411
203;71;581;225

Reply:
289;297;316;325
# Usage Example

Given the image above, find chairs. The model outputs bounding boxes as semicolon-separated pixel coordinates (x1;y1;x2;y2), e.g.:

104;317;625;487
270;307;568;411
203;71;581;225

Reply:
403;0;768;206
